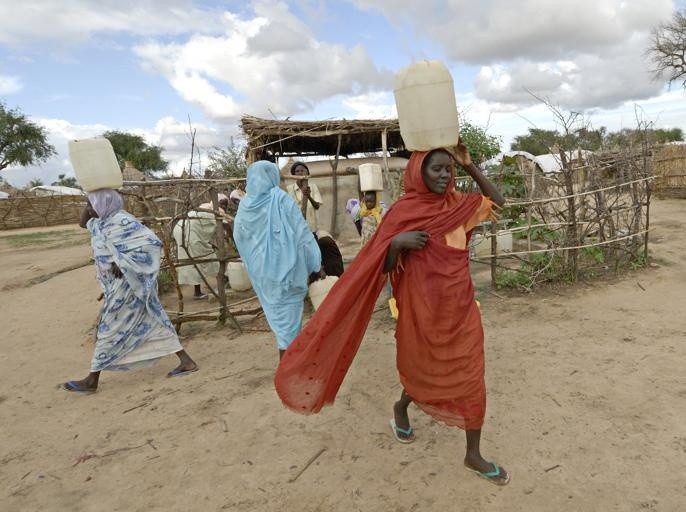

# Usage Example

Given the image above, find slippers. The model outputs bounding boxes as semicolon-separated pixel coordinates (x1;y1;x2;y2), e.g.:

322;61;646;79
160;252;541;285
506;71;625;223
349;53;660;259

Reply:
60;381;97;392
465;462;510;485
390;418;415;444
166;366;199;378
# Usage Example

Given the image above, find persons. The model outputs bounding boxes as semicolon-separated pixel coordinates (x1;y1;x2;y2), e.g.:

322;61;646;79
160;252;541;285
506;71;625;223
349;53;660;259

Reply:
345;199;387;236
228;189;245;205
356;174;389;250
171;194;230;300
56;187;199;394
233;161;332;363
284;161;321;234
384;140;512;484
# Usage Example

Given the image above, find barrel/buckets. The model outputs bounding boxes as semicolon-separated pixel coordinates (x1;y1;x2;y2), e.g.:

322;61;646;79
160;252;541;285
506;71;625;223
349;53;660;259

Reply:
357;163;385;193
472;233;492;257
226;257;252;293
495;229;514;260
67;137;124;194
391;58;460;152
308;275;339;312
